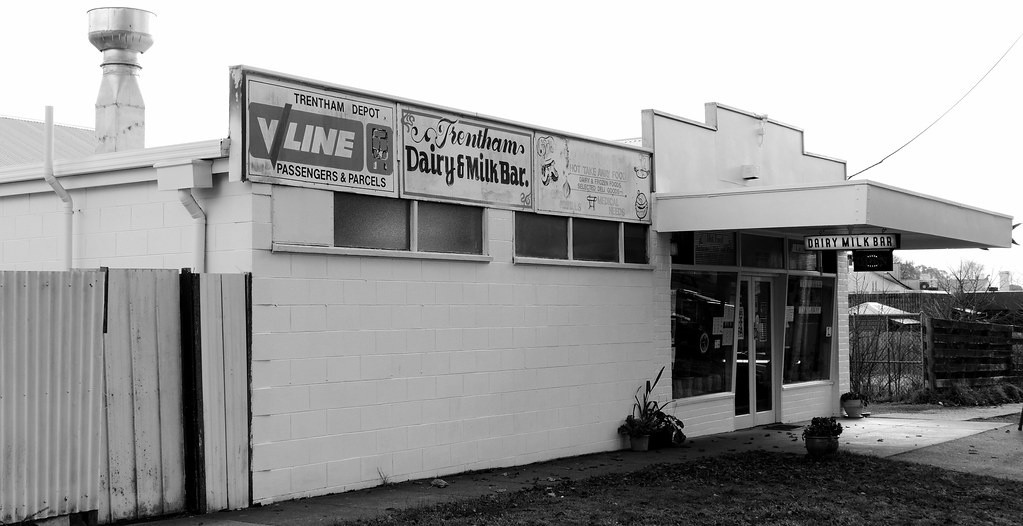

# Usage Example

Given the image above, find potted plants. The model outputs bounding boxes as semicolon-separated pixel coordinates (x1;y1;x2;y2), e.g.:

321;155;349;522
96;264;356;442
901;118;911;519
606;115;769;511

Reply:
615;366;686;451
802;415;843;454
840;392;869;418
